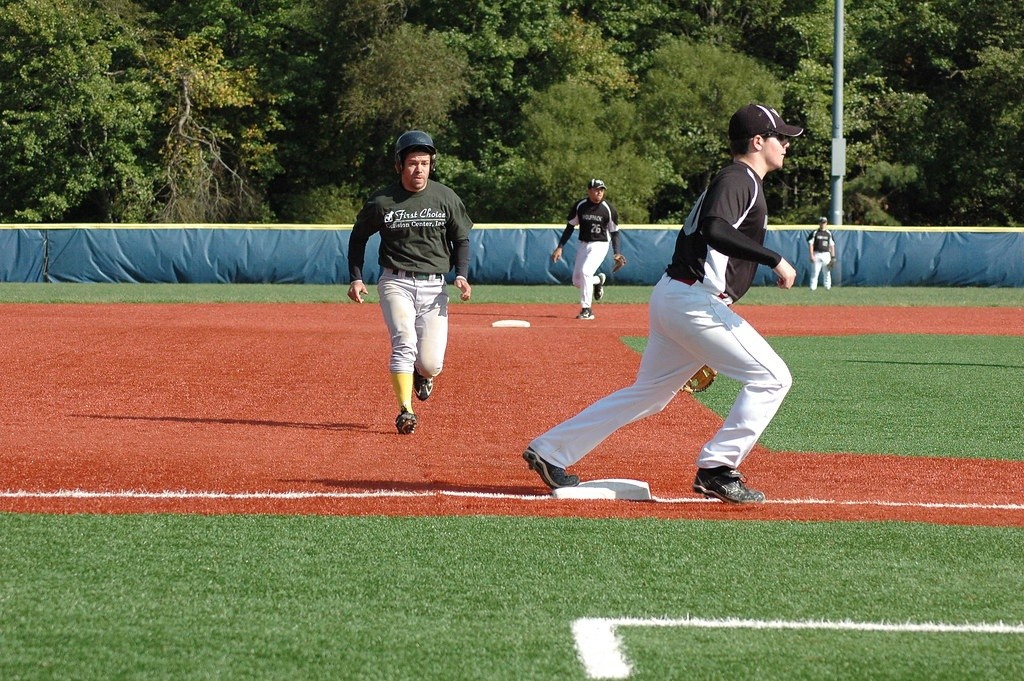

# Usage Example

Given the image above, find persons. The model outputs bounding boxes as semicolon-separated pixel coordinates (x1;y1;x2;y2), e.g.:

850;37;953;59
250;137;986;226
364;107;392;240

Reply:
521;103;804;504
347;132;474;435
806;216;836;291
552;179;626;320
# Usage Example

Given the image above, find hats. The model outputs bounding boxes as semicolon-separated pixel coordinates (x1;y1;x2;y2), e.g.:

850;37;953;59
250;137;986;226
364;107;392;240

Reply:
588;178;607;189
729;104;803;139
818;217;827;223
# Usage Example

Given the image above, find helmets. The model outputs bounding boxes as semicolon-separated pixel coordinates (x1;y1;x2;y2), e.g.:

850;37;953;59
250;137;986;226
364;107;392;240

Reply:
396;131;436;154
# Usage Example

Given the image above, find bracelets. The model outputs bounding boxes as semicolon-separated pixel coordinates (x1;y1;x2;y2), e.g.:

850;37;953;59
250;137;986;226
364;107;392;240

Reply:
832;256;836;258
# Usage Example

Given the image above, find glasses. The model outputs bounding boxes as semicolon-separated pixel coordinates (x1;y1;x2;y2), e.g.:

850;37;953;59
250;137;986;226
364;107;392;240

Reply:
761;133;790;142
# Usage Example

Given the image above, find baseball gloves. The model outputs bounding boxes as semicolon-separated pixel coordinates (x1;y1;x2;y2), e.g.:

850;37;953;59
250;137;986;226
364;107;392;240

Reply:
681;363;718;393
827;258;838;273
611;255;628;275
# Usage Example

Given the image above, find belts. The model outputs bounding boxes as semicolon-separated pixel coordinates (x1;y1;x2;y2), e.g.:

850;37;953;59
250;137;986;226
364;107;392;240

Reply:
391;268;442;280
673;277;729;307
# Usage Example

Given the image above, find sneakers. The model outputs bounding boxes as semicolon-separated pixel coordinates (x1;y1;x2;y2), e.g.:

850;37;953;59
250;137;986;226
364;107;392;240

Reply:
395;405;416;434
576;308;594;319
693;466;764;505
523;446;579;489
594;273;607;301
414;366;433;400
